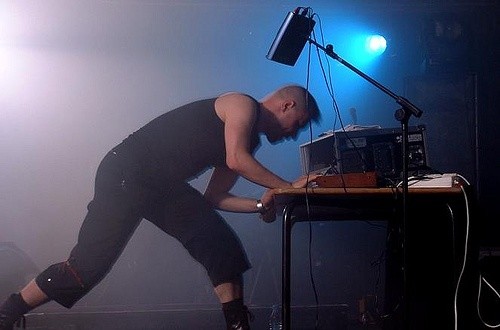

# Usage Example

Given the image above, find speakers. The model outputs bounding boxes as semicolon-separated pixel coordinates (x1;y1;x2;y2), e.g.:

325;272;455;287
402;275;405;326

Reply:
0;241;40;305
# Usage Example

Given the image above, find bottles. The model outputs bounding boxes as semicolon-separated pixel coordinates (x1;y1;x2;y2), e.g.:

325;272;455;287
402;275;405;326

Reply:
269;305;282;330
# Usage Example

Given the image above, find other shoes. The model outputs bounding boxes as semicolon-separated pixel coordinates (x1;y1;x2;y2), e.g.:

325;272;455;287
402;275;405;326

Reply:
0;293;28;330
220;304;255;330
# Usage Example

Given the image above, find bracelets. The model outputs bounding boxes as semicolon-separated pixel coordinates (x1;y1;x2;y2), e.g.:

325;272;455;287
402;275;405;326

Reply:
255;199;265;215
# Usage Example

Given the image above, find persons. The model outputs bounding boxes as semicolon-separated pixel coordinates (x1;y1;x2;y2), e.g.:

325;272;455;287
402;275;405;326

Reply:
1;85;321;330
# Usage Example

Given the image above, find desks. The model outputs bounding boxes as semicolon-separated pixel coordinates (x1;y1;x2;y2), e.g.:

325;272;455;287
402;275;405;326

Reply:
272;186;462;330
